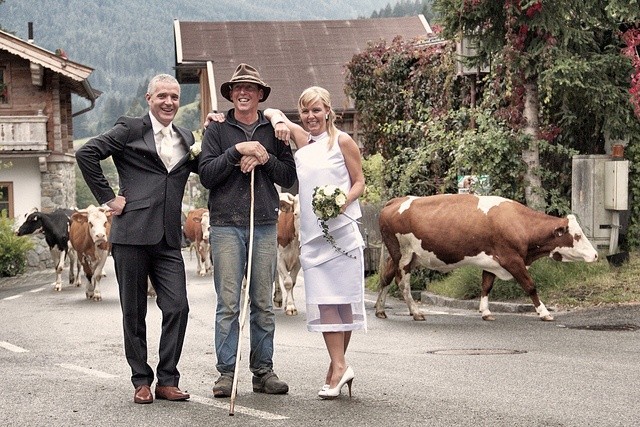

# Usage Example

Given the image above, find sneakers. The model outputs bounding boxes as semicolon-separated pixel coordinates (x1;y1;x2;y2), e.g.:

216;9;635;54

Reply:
252;372;288;395
213;373;237;398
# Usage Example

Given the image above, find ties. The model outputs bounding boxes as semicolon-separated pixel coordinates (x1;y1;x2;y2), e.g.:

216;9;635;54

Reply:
160;127;173;173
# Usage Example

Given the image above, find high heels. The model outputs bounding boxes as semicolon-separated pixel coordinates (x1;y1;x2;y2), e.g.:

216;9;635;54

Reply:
318;366;355;400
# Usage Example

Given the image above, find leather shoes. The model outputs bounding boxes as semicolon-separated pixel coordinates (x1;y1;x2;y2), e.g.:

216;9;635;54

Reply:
133;385;153;404
155;383;190;401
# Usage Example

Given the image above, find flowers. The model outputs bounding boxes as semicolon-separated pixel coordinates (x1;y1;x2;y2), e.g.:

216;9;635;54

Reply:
311;185;364;259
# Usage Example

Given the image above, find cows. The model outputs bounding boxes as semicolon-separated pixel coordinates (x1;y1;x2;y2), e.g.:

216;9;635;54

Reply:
15;205;82;293
66;205;113;301
186;206;213;278
272;190;302;316
371;192;600;323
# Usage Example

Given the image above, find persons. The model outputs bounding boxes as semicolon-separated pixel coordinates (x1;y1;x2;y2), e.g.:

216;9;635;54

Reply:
75;74;226;403
198;64;296;398
263;85;364;397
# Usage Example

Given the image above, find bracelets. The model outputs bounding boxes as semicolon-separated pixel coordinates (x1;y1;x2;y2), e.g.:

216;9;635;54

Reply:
271;114;285;128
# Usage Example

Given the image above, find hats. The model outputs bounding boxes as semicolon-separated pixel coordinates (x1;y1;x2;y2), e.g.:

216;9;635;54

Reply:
220;63;271;103
